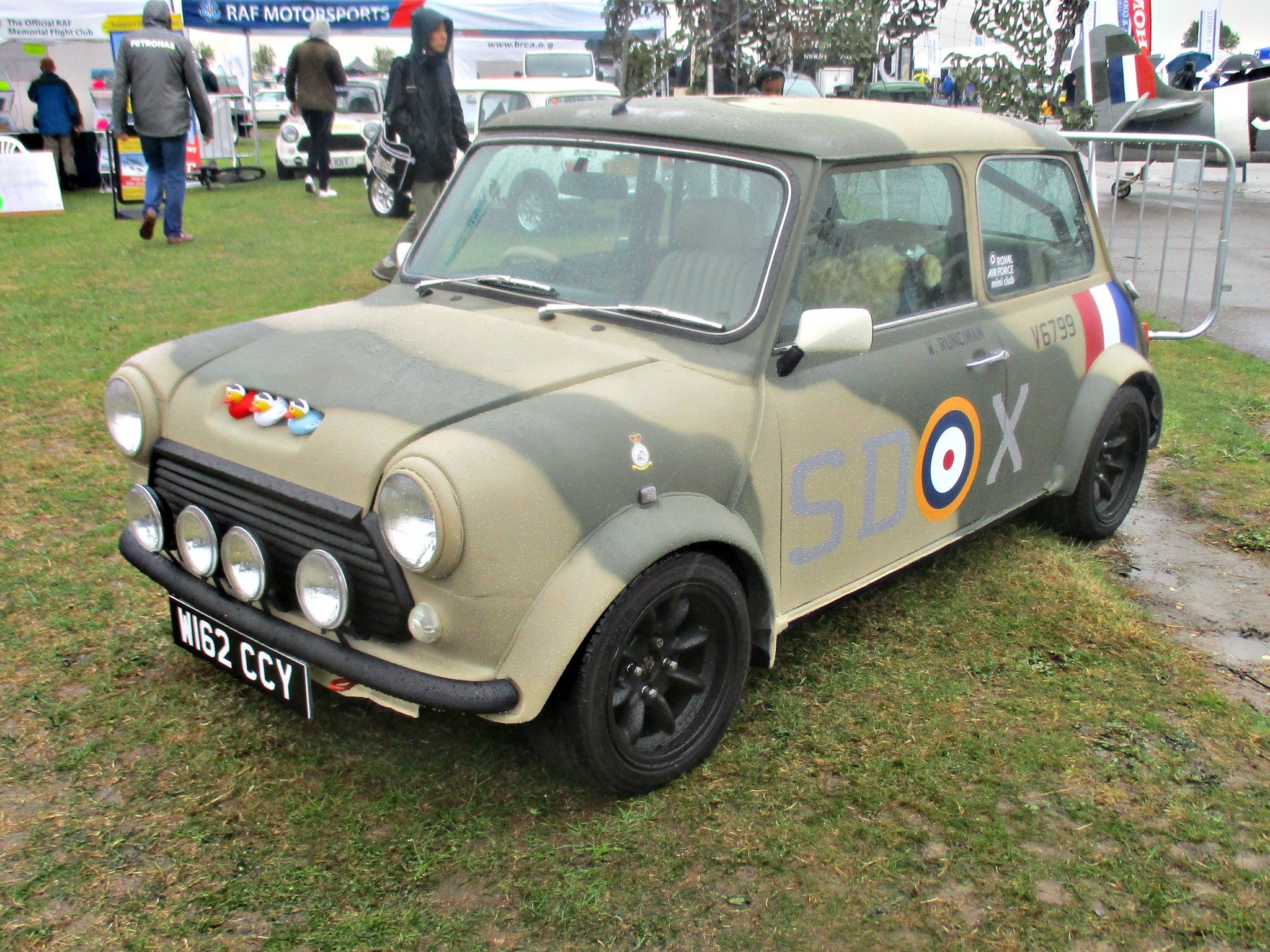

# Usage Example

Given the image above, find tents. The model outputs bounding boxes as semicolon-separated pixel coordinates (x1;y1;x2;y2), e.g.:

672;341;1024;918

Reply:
0;1;670;176
1154;46;1270;90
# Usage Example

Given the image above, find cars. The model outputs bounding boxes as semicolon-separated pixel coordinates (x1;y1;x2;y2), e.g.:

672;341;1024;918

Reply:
274;76;390;181
246;90;291;124
97;91;1165;800
366;77;644;233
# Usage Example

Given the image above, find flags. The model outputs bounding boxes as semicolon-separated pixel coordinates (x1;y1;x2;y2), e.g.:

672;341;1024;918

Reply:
1108;54;1156;105
1195;9;1221;78
1117;0;1151;57
927;32;942;80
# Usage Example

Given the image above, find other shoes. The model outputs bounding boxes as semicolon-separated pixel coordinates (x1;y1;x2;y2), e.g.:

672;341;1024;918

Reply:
306;175;316;194
166;231;193;245
373;259;398;282
318;188;336;197
139;209;157;240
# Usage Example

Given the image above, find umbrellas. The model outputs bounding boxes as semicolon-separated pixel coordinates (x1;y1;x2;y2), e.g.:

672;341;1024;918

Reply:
1216;51;1265;73
1165;51;1212;74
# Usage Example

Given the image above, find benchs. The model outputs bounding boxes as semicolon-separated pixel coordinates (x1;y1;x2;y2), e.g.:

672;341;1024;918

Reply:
812;218;1051;295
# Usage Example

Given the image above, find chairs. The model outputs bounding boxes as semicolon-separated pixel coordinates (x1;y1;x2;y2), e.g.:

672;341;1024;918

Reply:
350;97;376;114
640;198;762;322
0;135;28;154
802;217;941;320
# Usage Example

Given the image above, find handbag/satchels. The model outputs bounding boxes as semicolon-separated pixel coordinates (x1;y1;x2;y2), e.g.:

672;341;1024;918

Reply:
366;124;416;193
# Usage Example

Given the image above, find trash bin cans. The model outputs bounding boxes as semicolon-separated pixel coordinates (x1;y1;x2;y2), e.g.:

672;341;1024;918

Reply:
871;82;932;104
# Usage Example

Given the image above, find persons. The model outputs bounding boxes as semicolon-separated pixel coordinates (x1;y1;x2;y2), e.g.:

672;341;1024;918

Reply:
1202;73;1220;91
285;19;348;198
1176;61;1196;91
1062;71;1076;109
747;70;786;96
199;57;220;92
943;74;972;108
371;9;471;285
27;57;86;190
111;1;216;243
